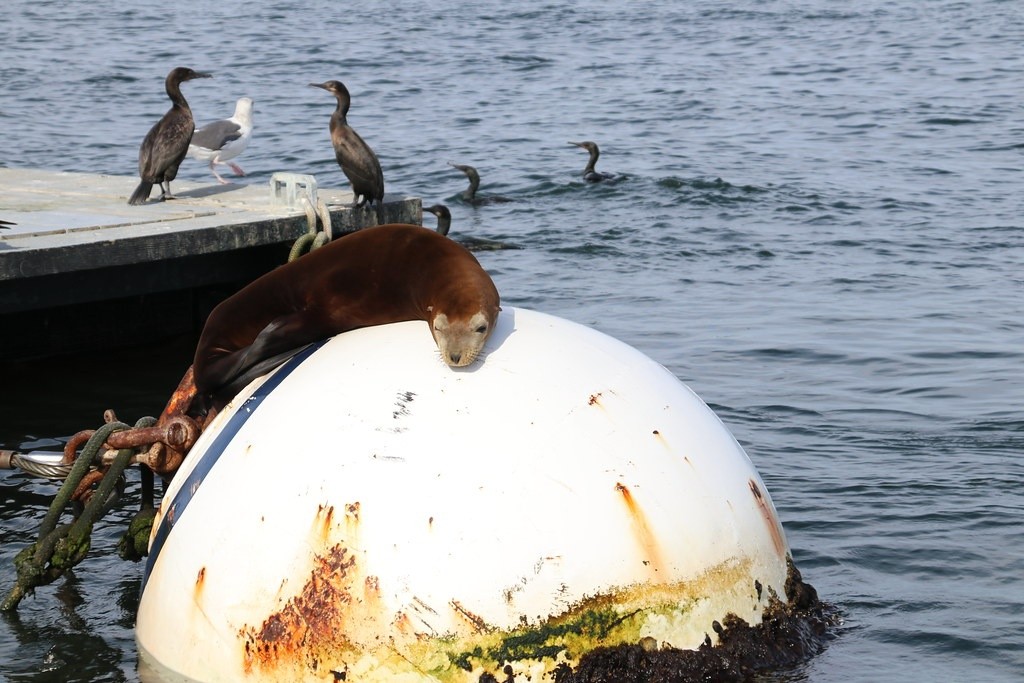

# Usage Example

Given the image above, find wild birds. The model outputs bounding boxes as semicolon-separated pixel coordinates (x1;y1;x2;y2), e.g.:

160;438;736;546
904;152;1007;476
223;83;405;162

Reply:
309;80;385;208
422;203;523;250
190;97;254;185
129;68;212;206
445;161;509;206
570;139;626;182
